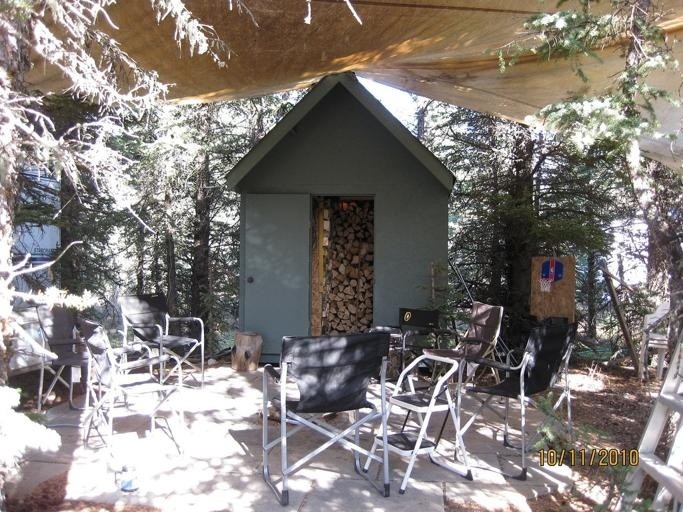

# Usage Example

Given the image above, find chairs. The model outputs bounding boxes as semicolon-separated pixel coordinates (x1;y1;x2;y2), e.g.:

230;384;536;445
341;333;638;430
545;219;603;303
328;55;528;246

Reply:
367;301;575;494
33;292;204;454
262;328;391;506
